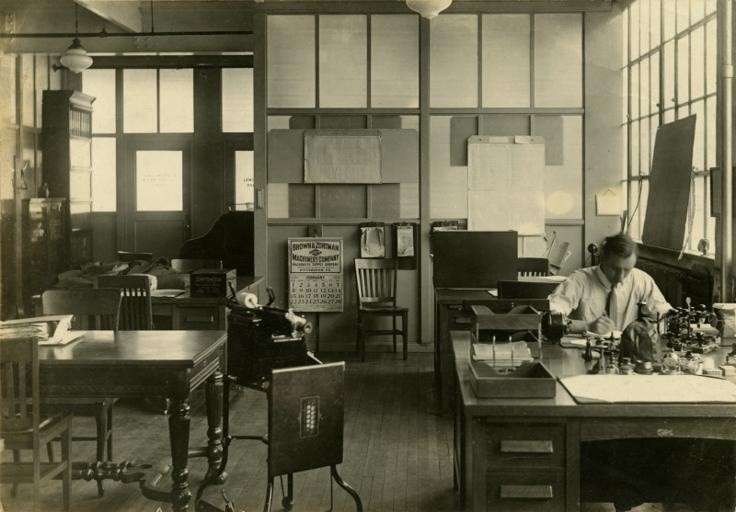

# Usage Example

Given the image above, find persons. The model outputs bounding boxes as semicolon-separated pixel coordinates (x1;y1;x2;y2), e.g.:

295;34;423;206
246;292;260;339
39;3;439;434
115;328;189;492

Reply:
546;231;680;510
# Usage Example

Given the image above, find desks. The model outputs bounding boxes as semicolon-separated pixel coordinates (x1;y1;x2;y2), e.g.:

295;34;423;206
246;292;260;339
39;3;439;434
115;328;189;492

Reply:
449;331;735;511
435;281;549;420
6;329;228;510
32;275;265;374
31;288;225;330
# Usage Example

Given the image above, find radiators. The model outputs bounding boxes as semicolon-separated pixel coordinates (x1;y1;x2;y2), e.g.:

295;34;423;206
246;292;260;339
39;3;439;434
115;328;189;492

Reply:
638;258;687;309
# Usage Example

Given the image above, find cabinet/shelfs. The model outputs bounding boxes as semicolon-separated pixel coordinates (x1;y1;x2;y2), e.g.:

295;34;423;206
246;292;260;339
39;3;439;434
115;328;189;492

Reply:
24;87;98;316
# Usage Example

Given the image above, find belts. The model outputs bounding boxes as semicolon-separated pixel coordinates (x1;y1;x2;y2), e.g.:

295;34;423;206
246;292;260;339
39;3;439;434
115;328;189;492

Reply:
606;284;619;326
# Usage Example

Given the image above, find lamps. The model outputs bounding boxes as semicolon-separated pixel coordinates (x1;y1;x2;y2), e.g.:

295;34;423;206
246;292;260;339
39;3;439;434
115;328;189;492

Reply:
406;0;453;21
59;0;94;75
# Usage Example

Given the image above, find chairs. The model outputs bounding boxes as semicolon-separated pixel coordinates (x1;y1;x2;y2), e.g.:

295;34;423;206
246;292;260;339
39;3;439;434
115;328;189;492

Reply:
11;287;123;498
93;275;153;331
353;257;410;364
2;335;73;511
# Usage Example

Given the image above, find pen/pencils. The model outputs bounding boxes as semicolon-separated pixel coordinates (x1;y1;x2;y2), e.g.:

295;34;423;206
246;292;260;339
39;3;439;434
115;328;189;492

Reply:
603;311;606;317
